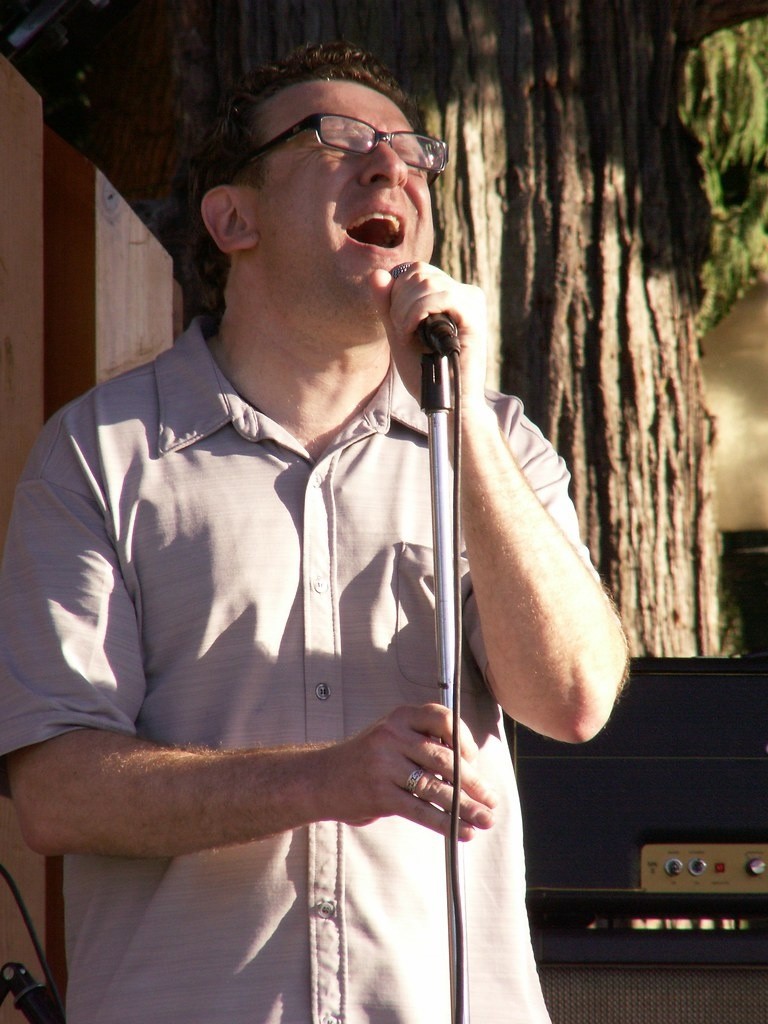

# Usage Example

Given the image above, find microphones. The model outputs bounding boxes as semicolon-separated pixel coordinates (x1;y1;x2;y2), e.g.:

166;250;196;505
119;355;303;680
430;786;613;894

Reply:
389;262;461;357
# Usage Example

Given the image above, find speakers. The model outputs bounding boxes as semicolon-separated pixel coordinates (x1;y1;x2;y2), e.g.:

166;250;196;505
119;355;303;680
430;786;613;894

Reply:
530;926;768;1024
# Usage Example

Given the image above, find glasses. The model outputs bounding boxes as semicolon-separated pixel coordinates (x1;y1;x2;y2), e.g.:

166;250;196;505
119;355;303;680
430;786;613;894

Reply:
220;113;447;186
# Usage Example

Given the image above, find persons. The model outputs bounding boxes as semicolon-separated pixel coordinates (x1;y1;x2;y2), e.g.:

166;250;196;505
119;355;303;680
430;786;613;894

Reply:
0;41;628;1024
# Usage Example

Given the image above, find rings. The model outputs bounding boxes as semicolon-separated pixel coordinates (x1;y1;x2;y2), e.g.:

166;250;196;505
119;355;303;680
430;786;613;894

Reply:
406;768;425;793
